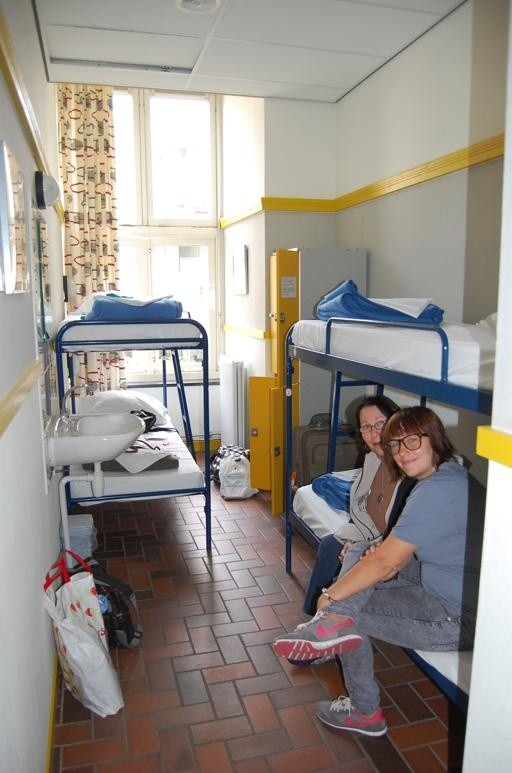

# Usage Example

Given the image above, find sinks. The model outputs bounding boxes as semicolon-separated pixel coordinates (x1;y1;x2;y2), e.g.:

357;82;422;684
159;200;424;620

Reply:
47;411;146;465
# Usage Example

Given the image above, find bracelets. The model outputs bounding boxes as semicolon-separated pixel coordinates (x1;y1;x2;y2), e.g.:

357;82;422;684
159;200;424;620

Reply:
321;587;337;603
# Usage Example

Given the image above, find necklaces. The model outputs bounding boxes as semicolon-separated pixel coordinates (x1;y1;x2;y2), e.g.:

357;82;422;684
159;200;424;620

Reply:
376;461;395;505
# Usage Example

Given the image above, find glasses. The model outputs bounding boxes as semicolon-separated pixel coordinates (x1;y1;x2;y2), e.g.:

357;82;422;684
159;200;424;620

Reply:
361;420;389;432
386;432;429;457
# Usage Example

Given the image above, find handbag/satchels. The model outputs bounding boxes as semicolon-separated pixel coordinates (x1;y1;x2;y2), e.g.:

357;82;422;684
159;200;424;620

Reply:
209;443;250;484
39;552;123;720
67;557;142;650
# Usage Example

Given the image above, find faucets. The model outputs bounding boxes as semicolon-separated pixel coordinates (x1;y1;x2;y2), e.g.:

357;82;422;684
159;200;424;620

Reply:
60;383;89;425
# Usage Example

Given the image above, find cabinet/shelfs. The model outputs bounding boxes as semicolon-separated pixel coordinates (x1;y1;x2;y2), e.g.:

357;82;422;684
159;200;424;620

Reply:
248;247;368;517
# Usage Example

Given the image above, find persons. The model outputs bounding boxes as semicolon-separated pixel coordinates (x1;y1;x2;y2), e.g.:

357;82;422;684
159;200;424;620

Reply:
271;405;484;740
287;394;416;667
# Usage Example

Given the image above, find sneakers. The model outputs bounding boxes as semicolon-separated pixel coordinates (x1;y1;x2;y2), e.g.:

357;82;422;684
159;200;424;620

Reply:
316;696;389;737
273;609;364;660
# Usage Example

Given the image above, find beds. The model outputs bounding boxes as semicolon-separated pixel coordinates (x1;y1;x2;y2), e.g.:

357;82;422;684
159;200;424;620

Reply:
55;313;211;552
285;316;496;716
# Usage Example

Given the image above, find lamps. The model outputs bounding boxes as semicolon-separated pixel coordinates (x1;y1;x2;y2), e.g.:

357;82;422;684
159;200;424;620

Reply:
35;171;60;209
63;276;76;302
